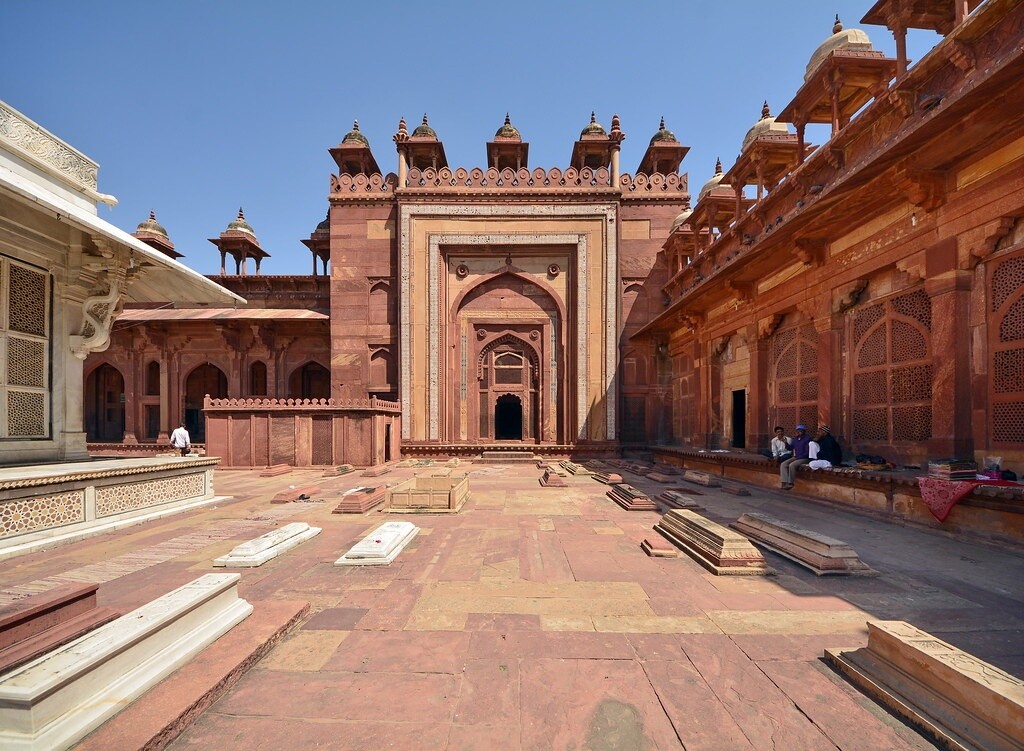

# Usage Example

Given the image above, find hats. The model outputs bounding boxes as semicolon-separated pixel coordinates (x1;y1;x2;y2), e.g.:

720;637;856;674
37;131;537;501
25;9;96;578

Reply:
796;425;806;430
819;425;832;434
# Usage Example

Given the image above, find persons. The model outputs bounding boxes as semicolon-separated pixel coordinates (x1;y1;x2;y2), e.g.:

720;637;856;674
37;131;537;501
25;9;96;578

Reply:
809;426;843;471
768;426;793;468
779;425;812;489
169;422;191;456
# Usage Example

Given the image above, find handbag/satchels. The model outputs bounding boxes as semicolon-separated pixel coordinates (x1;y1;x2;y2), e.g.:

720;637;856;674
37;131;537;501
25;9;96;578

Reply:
982;456;1003;480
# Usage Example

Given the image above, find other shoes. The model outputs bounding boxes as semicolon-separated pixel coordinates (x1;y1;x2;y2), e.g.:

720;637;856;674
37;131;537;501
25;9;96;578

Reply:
806;462;810;467
780;482;793;489
